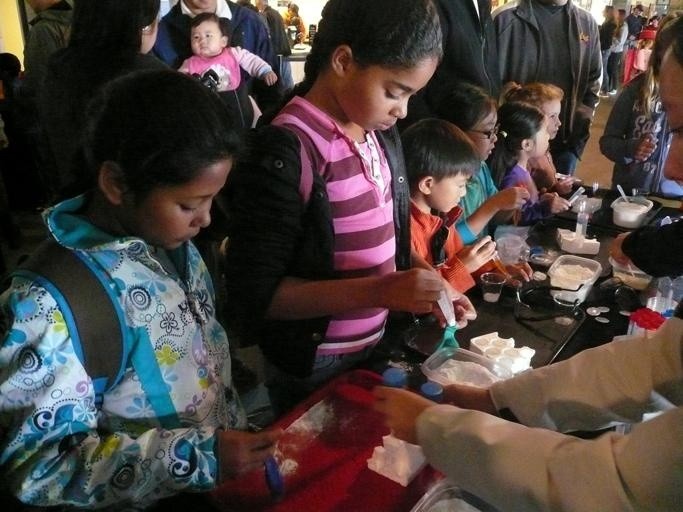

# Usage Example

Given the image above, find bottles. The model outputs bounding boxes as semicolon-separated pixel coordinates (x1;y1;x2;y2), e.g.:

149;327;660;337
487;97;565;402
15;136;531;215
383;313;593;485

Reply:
575;201;589;238
567;186;586;203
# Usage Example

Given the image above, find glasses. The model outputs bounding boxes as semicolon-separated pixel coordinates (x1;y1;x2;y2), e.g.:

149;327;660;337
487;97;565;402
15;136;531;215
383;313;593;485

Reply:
514;282;585;322
470;123;501;139
598;276;642;311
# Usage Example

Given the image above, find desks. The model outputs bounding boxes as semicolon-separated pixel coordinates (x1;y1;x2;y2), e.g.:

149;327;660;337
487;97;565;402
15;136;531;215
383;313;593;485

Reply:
372;183;683;439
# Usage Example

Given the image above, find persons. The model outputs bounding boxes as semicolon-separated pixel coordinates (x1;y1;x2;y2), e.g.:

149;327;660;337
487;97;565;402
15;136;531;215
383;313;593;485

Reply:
0;0;304;202
401;81;573;296
599;10;682;198
373;15;683;512
224;0;478;419
598;5;659;98
432;0;604;175
0;68;286;512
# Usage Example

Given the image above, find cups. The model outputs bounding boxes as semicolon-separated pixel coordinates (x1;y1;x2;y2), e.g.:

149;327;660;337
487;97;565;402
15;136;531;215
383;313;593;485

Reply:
480;273;507;303
496;237;523;265
